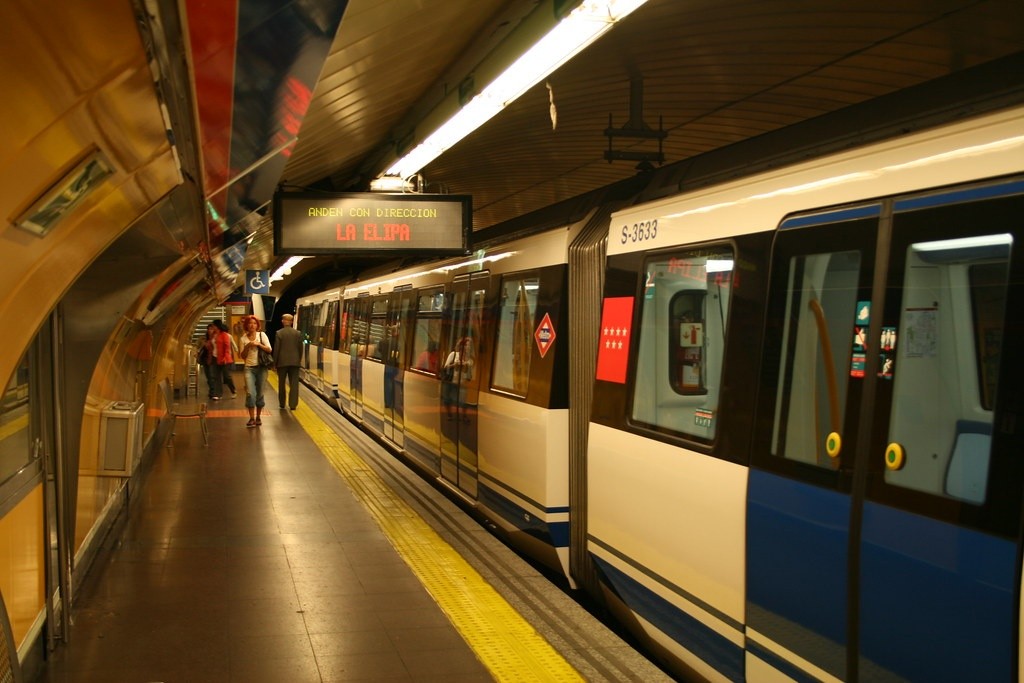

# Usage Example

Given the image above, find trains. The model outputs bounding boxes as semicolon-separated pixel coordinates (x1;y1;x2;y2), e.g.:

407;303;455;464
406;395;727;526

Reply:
290;101;1024;683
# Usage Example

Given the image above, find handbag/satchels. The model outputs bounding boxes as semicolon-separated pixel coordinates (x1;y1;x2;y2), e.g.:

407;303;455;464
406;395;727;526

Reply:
196;345;209;365
258;332;274;369
440;351;456;383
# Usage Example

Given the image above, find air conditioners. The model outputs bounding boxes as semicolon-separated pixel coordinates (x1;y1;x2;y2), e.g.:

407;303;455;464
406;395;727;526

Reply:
95;400;145;477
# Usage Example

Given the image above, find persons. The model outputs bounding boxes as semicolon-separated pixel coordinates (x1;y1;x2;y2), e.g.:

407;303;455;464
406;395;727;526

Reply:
351;334;374;391
445;338;474;424
383;325;400;408
191;319;239;400
416;340;438;372
238;315;272;427
274;314;304;411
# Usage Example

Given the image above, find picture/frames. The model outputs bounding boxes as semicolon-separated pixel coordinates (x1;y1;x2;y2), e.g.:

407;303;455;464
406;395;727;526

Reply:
14;148;120;240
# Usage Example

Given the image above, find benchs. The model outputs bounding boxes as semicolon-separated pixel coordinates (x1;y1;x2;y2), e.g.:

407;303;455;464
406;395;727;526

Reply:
158;376;209;449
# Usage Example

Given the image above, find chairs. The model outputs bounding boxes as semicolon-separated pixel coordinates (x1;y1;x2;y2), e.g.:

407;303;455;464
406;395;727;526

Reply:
942;419;993;504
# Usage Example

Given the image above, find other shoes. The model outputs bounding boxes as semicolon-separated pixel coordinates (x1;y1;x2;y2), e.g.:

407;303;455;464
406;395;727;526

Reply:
213;396;222;400
256;418;261;425
245;419;256;427
231;389;237;398
447;414;453;421
463;416;470;424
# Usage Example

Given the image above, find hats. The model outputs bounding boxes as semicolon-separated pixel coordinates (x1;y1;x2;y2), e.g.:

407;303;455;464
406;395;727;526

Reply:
282;314;293;321
